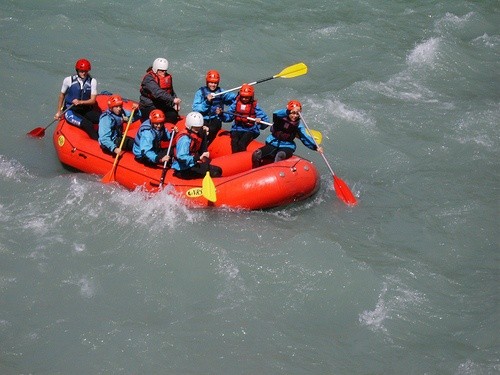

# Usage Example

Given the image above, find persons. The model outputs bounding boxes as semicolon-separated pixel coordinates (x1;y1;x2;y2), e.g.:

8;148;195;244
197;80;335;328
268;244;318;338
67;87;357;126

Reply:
192;70;248;148
224;85;270;154
138;57;183;124
132;109;179;168
98;95;141;156
252;99;323;169
54;58;103;141
171;112;222;180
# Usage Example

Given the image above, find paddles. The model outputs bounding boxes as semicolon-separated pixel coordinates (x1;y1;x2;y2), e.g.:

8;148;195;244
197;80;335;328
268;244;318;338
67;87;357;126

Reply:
211;61;307;101
295;108;357;205
102;107;135;185
218;109;323;148
146;127;178;201
174;98;180;126
27;101;80;138
199;133;217;202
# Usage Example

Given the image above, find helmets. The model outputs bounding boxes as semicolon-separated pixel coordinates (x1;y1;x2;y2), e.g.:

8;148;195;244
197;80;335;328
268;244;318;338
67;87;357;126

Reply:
287;100;301;112
240;84;253;96
206;70;220;83
149;109;166;123
76;59;91;71
184;112;204;131
152;57;168;74
107;95;123;110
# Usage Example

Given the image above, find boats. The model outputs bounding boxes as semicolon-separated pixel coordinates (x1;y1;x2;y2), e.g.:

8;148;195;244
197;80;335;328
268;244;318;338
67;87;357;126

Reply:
52;91;318;210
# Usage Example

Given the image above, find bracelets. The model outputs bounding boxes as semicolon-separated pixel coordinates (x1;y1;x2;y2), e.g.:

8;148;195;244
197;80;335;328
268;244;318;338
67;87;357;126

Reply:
56;113;59;116
